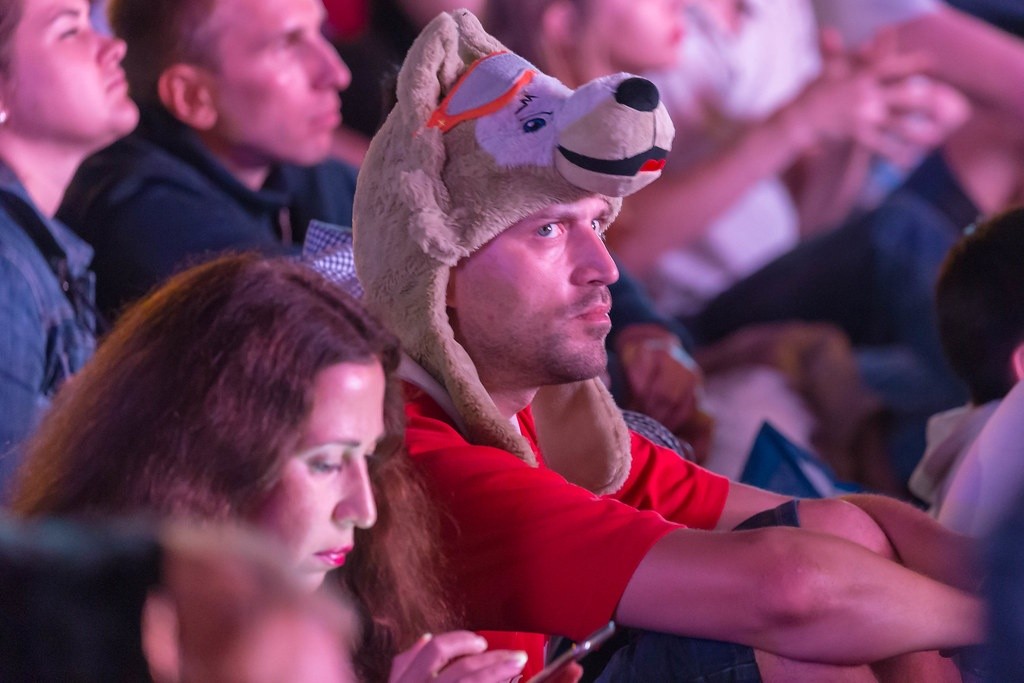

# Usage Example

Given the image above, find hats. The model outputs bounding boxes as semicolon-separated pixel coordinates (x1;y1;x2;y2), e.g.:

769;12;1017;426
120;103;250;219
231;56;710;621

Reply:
351;9;675;498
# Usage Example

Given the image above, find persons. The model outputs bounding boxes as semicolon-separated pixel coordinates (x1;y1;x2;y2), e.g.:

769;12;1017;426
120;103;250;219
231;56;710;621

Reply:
20;250;587;683
0;0;1024;683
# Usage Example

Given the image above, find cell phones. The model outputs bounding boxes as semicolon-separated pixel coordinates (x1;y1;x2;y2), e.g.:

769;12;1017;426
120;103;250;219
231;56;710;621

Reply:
526;623;615;683
